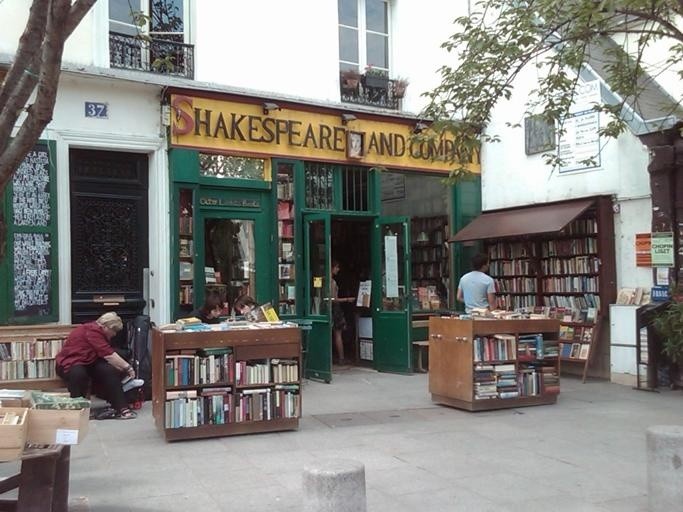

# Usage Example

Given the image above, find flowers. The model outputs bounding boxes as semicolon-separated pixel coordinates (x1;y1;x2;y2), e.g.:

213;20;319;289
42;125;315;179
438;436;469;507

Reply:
361;63;389;78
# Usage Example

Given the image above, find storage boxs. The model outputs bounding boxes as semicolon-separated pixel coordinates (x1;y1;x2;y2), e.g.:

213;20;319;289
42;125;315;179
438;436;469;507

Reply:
0;387;92;460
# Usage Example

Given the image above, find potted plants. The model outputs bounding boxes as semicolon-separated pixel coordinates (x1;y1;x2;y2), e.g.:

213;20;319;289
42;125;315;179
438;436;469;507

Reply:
390;75;411;97
644;279;683;387
340;69;360;89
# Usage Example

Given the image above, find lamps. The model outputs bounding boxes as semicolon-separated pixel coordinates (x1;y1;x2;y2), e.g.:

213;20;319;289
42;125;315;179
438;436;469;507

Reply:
262;102;281;115
414;121;429;134
341;113;357;125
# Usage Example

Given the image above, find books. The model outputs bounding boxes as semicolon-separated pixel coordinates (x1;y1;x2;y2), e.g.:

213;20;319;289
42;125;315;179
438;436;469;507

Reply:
355;278;373;307
256;302;279;322
179;216;192;305
163;321;301;429
274;175;295;300
0;338;65;424
474;219;598;399
410;219;450;309
614;287;650;306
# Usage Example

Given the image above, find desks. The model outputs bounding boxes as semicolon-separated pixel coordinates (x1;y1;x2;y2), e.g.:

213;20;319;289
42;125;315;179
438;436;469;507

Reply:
410;319;430;328
0;442;70;512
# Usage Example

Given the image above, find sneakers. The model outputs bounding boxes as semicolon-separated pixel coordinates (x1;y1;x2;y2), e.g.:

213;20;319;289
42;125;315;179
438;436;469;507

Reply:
121;410;137;419
97;407;117;420
122;379;145;392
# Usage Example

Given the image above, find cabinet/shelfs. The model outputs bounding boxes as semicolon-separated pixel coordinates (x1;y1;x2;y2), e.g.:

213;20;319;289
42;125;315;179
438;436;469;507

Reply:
150;322;303;442
446;196;618;383
427;314;562;409
0;324;84;390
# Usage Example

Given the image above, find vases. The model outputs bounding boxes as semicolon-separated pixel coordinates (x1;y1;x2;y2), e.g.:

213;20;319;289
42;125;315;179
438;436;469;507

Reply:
361;75;389;88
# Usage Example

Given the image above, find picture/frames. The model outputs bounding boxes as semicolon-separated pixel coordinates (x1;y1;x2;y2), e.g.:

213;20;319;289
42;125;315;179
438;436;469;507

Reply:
346;129;366;160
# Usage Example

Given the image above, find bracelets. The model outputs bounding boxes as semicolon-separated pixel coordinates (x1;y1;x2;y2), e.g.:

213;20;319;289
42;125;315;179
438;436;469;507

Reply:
124;364;134;373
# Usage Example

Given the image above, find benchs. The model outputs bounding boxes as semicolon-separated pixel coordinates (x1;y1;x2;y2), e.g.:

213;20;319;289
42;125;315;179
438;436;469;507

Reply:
412;340;428;374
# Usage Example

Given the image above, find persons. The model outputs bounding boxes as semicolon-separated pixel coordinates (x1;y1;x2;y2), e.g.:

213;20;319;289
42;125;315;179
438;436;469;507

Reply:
55;309;137;419
331;261;356;365
234;295;260;319
192;297;225;323
457;253;498;316
